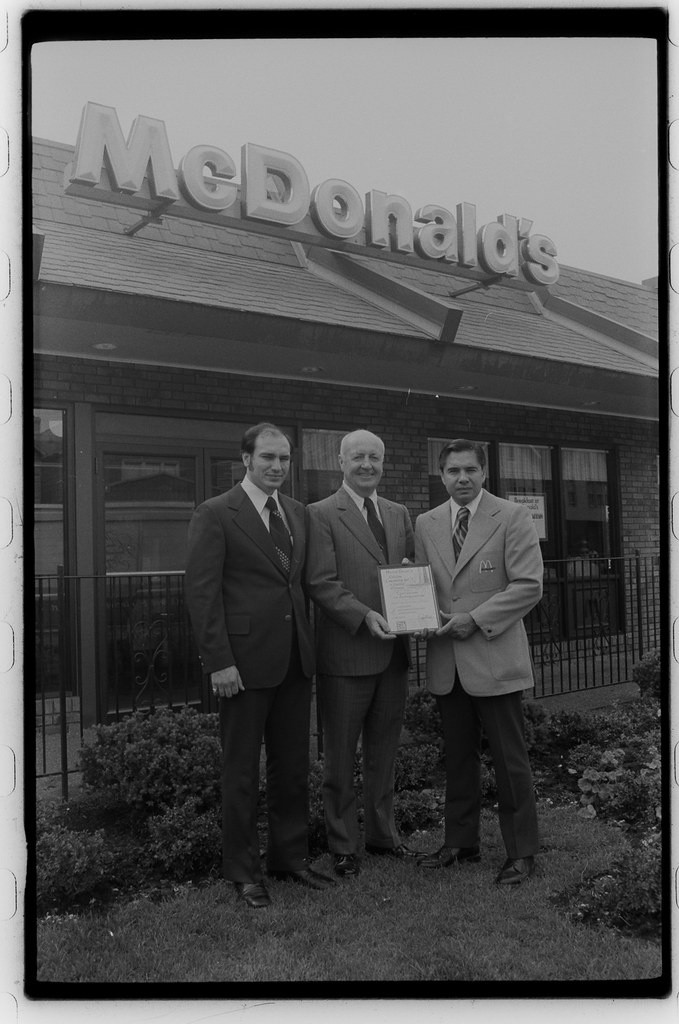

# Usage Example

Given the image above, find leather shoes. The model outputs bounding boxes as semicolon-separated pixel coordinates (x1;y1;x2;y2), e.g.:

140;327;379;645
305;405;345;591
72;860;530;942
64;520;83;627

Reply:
266;864;338;890
365;844;428;861
417;841;481;868
333;854;359;877
233;881;272;908
493;855;534;884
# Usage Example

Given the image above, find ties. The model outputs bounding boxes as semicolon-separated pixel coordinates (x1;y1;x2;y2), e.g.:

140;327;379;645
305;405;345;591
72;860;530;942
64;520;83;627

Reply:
364;497;389;566
265;496;293;572
452;507;470;565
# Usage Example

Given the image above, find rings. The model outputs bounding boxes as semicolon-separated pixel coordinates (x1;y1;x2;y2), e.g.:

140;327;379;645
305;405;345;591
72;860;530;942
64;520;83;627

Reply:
212;688;216;692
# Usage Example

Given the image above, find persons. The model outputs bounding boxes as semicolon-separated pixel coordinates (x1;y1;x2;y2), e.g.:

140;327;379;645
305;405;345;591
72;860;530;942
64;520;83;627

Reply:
303;429;416;879
185;424;328;907
414;438;544;886
